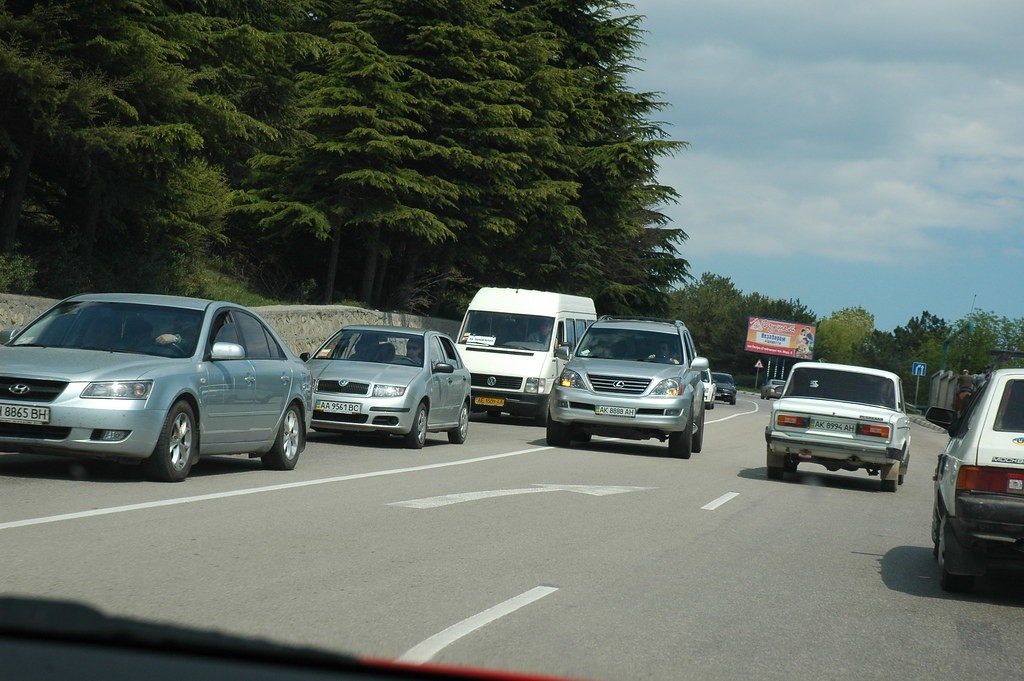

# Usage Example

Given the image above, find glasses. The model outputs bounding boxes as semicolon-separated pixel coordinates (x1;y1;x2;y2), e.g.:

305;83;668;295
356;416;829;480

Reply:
656;345;667;351
405;345;419;350
540;323;549;327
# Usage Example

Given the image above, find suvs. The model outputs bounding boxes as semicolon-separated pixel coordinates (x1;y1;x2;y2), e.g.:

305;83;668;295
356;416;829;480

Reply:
546;313;709;460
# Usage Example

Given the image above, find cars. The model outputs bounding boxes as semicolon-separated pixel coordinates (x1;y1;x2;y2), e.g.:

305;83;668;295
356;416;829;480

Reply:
925;368;1024;591
761;379;786;400
701;368;717;409
711;372;737;405
0;293;313;483
300;325;471;448
764;362;911;492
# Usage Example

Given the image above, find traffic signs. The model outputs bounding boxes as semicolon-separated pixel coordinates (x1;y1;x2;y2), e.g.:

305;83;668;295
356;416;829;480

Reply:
912;362;927;376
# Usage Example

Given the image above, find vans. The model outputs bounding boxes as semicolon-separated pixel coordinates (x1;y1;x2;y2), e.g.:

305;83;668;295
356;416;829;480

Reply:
453;287;598;427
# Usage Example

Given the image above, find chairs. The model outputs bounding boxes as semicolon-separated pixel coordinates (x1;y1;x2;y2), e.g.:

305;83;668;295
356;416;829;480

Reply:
608;337;633;359
494;319;523;345
349;339;373;362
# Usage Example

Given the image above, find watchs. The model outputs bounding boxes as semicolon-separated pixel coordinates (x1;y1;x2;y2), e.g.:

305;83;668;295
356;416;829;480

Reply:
174;335;182;344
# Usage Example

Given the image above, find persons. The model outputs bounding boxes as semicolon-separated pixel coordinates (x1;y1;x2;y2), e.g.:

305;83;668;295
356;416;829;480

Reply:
407;338;423;365
525;319;558;349
152;308;212;362
648;340;679;364
956;369;974;416
796;327;814;354
